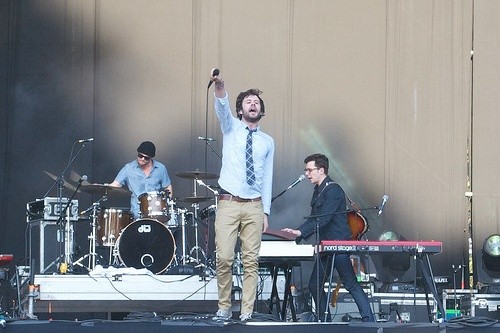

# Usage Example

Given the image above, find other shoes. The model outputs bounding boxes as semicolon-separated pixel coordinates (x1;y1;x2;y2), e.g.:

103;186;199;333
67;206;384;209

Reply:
317;314;332;321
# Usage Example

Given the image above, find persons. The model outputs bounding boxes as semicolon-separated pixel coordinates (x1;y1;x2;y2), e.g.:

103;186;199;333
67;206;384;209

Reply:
281;153;375;324
106;141;173;221
210;68;275;321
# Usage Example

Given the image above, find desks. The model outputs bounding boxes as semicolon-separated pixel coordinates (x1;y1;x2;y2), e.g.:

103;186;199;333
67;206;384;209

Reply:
259;257;314;322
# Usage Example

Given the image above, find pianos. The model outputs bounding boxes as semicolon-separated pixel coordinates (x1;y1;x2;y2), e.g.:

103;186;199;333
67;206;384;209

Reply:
257;230;316;322
314;240;444;322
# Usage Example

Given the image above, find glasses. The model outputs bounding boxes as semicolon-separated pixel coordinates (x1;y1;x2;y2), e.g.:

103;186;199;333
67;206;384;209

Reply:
304;168;318;172
138;152;151;160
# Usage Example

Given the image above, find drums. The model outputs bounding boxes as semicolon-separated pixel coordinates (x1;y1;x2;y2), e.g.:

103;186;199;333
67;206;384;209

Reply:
165;199;179;228
96;207;135;247
115;218;177;275
137;190;171;223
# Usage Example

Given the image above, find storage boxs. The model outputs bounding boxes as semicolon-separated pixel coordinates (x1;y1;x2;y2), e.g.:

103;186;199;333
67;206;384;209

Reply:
26;221;73;274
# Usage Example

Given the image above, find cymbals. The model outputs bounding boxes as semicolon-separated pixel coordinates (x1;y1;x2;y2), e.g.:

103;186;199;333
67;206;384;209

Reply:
80;184;132;198
175;171;220;179
43;170;81;194
70;170;91;186
183;195;212;203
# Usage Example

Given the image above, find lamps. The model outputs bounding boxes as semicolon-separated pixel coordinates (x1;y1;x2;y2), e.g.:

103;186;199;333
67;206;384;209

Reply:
378;230;410;271
480;234;500;271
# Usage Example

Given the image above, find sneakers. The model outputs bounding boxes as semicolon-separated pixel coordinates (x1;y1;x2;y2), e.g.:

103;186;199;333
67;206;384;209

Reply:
213;309;233;320
239;312;252;321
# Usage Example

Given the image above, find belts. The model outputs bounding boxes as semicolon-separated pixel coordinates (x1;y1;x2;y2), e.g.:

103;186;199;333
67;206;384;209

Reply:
220;194;261;202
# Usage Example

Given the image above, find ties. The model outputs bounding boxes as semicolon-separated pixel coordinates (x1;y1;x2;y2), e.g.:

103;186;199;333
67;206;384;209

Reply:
245;127;257;186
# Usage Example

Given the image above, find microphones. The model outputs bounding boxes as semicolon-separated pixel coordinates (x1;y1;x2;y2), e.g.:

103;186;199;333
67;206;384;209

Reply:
378;195;389;215
76;175;88;183
285;174;306;191
198;136;216;142
77;138;94;142
207;68;220;88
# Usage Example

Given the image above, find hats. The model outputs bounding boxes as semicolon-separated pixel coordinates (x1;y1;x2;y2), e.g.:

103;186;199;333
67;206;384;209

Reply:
137;141;156;157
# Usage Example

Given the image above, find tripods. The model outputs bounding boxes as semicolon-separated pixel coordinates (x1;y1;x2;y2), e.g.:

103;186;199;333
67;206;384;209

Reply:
186;175;213;268
37;143;108;275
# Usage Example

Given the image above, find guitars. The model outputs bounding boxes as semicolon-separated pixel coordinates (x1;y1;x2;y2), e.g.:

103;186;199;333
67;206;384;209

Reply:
330;210;369;308
